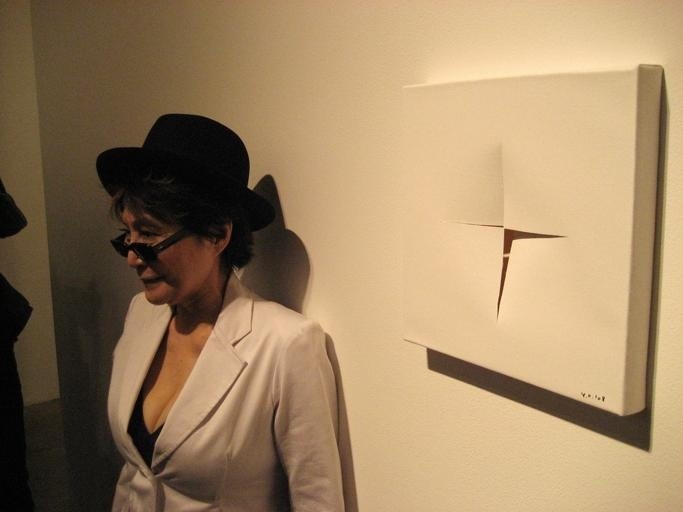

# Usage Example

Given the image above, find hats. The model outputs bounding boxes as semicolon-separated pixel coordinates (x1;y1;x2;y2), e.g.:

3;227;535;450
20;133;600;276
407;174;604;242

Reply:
95;113;276;232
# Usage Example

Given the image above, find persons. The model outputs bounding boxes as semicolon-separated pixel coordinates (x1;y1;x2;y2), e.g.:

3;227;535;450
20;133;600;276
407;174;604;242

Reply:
0;178;39;512
96;114;345;512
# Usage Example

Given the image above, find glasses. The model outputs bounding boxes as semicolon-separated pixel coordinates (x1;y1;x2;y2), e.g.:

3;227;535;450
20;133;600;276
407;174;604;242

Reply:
110;226;192;265
